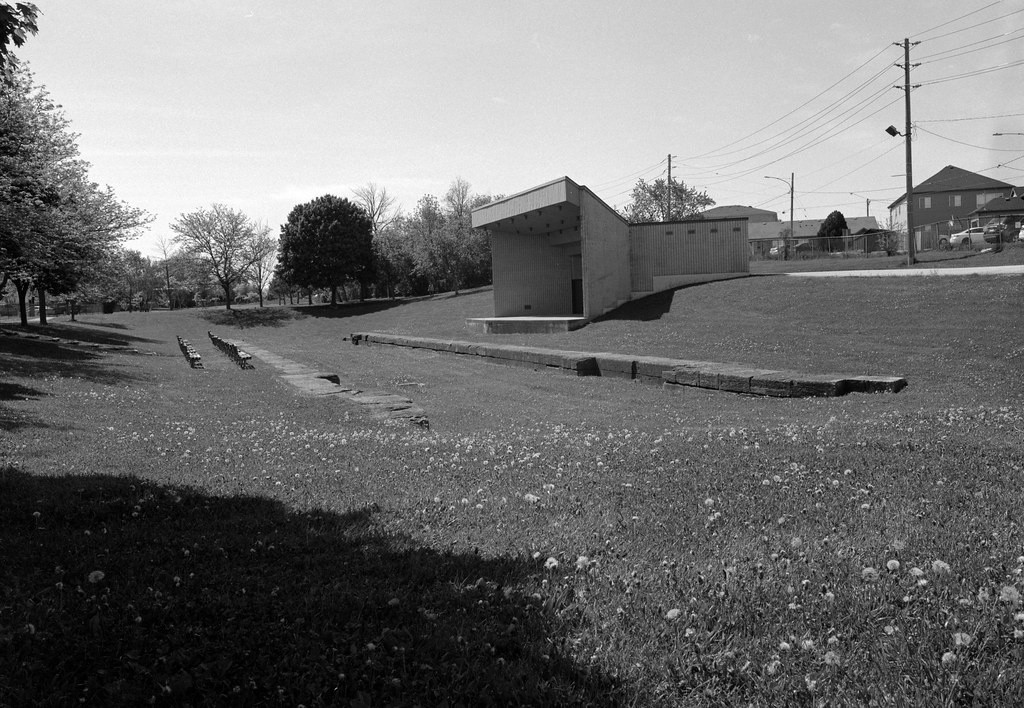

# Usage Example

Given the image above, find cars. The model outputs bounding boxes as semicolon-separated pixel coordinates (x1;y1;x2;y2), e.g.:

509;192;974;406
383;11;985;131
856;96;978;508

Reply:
770;245;786;259
950;227;986;248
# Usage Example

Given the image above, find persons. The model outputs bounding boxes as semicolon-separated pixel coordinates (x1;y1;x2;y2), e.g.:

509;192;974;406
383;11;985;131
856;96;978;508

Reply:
427;281;434;293
320;290;329;303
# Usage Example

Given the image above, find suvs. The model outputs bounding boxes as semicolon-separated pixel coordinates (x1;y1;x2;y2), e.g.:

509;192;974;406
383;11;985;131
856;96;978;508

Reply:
982;214;1024;242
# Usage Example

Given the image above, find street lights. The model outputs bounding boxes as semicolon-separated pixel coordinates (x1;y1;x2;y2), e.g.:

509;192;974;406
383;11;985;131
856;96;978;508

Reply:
763;173;795;258
884;124;916;266
